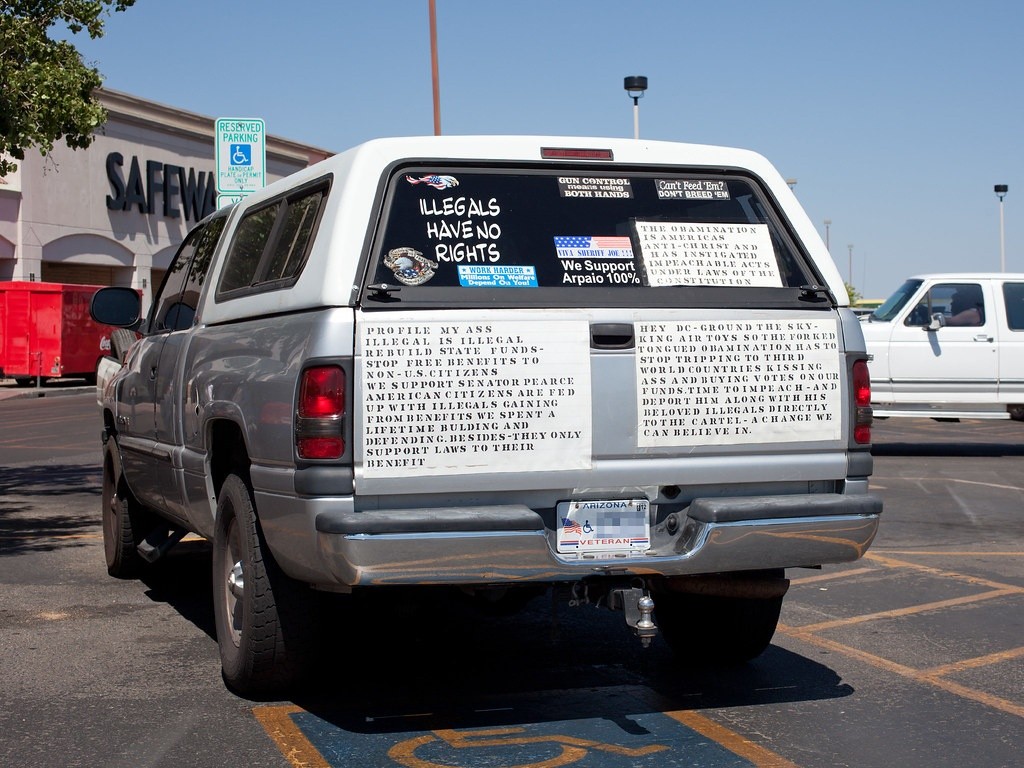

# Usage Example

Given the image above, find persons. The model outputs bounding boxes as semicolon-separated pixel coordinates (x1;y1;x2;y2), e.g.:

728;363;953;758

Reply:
945;293;985;326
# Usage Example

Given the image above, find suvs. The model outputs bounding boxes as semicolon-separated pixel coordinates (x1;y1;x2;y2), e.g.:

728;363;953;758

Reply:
90;134;884;689
852;272;1024;422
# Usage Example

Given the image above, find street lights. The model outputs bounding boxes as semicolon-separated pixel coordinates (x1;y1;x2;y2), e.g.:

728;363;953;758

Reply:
622;75;649;140
786;178;798;196
822;219;833;253
846;242;854;290
992;183;1009;272
